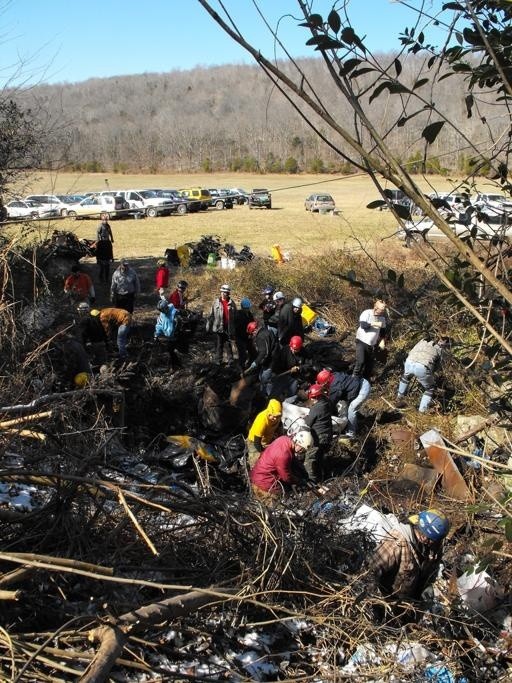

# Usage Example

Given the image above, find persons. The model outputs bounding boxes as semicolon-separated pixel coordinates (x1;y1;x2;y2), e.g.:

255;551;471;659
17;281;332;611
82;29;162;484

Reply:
366;508;450;624
269;336;316;396
316;369;370;437
109;258;140;315
154;259;169;300
302;384;334;484
397;338;442;413
352;299;387;376
169;280;196;309
266;291;289;336
96;234;114;283
96;216;114;242
250;431;314;507
246;322;283;400
246;399;281;468
64;265;95;305
206;284;237;366
258;288;276;325
279;298;305;345
153;300;183;371
234;298;255;371
89;307;132;355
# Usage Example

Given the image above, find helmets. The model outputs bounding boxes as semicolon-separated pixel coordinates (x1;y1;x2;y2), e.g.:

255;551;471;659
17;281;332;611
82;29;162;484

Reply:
177;280;187;288
75;371;89;385
71;267;81;274
155;259;165;265
419;508;451;541
373;300;387;311
293;297;303;309
120;258;130;265
293;432;314;450
157;300;167;310
240;299;250;308
262;288;274;293
89;309;100;317
289;336;303;351
77;303;88;311
273;291;286;300
318;370;333;386
247;321;261;333
308;385;324;397
219;285;231;293
100;214;110;220
268;398;282;417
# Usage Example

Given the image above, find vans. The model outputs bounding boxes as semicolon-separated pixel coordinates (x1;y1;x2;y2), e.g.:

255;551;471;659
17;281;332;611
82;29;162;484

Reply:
100;190;127;200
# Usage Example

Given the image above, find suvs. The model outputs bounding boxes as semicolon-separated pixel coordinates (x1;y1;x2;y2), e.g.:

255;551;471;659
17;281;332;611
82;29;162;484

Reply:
398;211;476;248
440;195;463;208
250;189;272;208
380;188;411;211
180;186;211;210
208;189;234;209
438;194;505;216
26;195;79;218
155;190;201;214
116;190;175;217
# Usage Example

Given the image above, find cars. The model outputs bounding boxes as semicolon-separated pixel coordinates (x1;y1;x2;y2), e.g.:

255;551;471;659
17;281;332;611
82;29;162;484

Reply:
3;200;57;220
66;196;129;220
305;193;335;212
